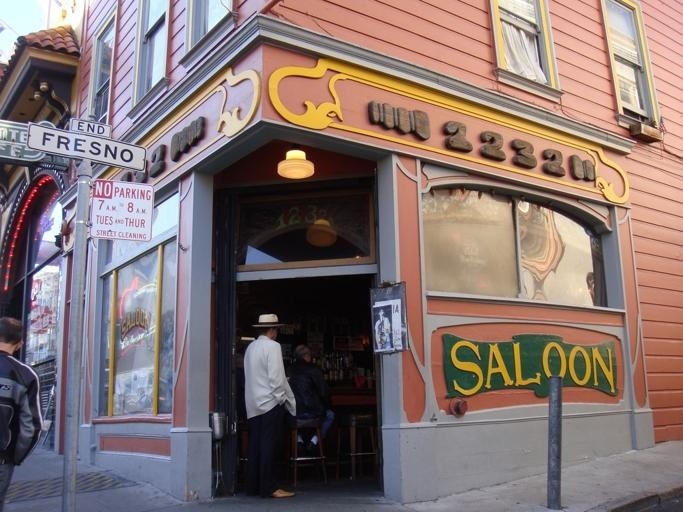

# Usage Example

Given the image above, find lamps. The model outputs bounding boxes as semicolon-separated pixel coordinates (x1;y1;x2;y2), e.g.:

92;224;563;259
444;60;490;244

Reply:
276;144;315;180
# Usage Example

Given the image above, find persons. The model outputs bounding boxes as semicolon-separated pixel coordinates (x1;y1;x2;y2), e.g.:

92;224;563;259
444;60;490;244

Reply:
0;317;42;511
289;344;335;456
241;313;300;499
374;309;391;349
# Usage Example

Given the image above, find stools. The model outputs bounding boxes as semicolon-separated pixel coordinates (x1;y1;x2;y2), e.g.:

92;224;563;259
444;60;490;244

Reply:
290;414;379;493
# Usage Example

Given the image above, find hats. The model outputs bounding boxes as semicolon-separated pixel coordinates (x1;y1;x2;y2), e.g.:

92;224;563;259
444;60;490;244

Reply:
295;345;310;360
252;314;283;327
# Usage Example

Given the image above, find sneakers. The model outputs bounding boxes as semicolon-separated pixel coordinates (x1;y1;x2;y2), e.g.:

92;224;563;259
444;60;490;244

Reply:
305;441;320;450
297;441;304;457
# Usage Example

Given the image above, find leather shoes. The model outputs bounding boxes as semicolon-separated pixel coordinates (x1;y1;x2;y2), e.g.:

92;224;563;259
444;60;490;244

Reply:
272;489;295;498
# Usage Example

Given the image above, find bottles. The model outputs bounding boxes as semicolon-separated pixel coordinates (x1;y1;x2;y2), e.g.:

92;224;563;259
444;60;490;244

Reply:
283;352;351;383
